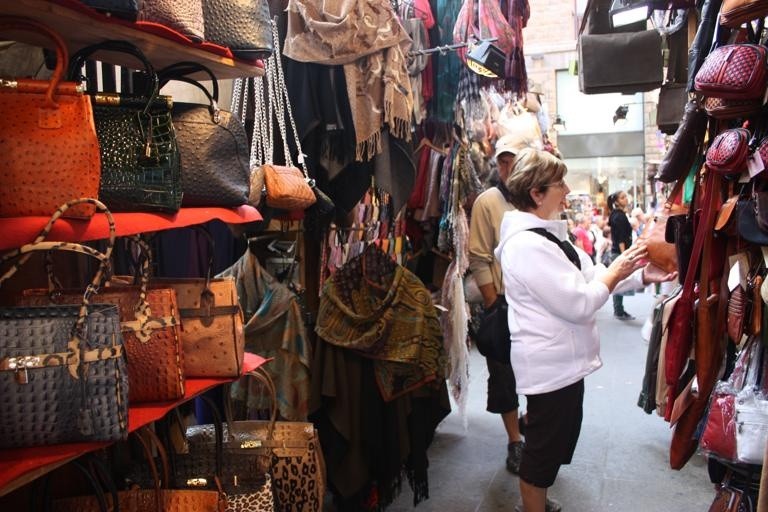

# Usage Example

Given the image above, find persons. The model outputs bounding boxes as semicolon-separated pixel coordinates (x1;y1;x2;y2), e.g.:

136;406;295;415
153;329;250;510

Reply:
626;200;663;298
494;151;677;512
470;135;529;473
608;191;636;319
563;193;612;268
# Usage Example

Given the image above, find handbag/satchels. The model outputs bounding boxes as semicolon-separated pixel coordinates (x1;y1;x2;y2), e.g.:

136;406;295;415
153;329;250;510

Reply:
229;76;263;205
124;393;279;512
657;30;691;134
576;0;664;94
655;101;704;183
634;137;705;273
265;20;315;210
708;486;753;512
20;234;184;403
694;43;768;98
706;128;749;173
727;265;749;345
696;168;728;398
665;169;715;386
736;332;768;465
52;426;228;512
755;137;768;179
705;82;768;119
0;17;99;220
745;276;762;336
666;171;702;285
186;366;326;512
637;287;685;417
112;224;245;379
204;1;272;60
752;179;768;232
65;40;182;213
0;198;129;449
670;379;712;470
720;0;767;28
701;340;755;461
272;21;334;236
139;0;204;43
714;195;741;232
156;62;250;208
737;201;768;245
86;0;138;22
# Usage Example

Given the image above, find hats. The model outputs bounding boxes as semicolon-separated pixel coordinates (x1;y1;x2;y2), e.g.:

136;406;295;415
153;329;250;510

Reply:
495;135;529;157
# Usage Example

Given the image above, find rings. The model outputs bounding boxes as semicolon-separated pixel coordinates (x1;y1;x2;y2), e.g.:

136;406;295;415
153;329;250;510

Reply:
628;254;634;261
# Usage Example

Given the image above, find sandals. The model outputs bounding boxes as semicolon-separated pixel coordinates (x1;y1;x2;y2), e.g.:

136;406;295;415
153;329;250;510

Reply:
515;496;561;512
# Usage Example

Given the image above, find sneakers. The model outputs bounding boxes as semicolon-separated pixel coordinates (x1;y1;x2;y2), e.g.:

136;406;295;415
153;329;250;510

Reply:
518;415;526;436
506;441;525;475
614;312;634;320
